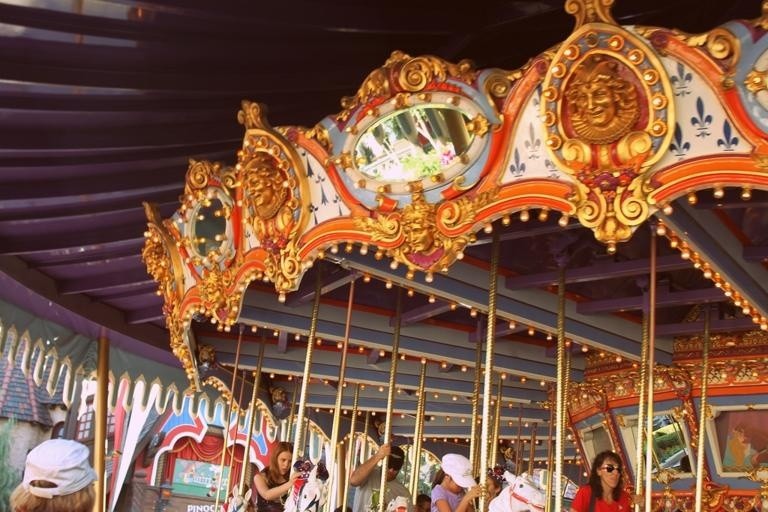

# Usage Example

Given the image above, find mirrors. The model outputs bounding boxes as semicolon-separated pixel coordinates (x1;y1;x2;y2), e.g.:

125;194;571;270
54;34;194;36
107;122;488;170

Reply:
185;189;233;265
343;91;489;195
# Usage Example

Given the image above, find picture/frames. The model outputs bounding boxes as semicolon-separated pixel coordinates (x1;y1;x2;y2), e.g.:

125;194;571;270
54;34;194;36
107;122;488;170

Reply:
615;407;695;483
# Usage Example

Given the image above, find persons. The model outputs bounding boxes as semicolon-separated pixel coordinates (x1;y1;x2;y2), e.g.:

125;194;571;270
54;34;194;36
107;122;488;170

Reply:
430;453;486;512
412;494;432;512
563;62;640;145
398;203;437;252
251;442;299;512
568;449;633;512
7;434;95;512
347;440;415;512
473;470;503;512
221;483;255;512
239;154;284;221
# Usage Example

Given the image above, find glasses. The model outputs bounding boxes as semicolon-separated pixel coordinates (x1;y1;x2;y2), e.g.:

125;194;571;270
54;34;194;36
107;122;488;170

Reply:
388;464;399;470
600;464;621;473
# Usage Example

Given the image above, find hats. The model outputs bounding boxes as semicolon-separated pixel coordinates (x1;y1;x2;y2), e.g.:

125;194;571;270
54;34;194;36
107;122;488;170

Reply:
23;438;97;499
388;446;404;466
440;453;477;488
